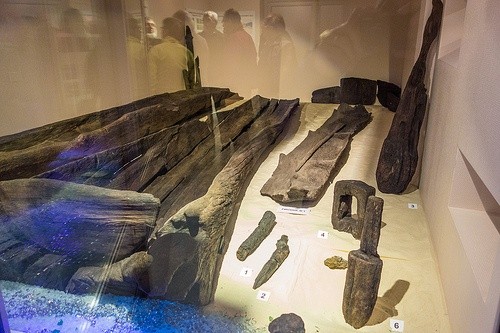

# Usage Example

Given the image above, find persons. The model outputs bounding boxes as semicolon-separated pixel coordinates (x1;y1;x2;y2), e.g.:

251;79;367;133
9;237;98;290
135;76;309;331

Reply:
0;7;298;136
